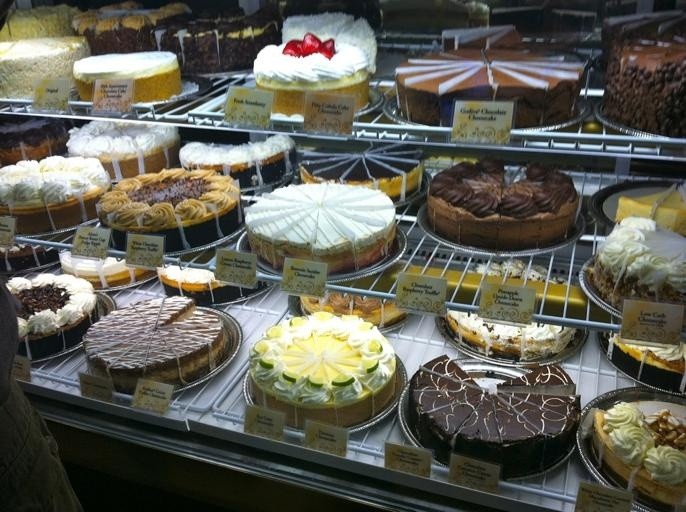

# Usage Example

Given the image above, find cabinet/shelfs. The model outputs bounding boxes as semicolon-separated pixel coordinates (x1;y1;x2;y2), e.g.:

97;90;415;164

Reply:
1;76;685;511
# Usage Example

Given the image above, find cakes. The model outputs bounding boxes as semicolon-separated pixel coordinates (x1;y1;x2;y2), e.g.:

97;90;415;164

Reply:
427;159;581;252
82;295;232;395
587;216;685;311
179;132;296;189
440;258;582;362
95;168;244;253
406;354;581;477
599;312;685;394
615;183;685;236
394;48;590;127
5;273;100;363
1;232;72;271
71;1;193;57
0;36;91;101
59;245;156;289
9;5;78;42
0;155;112;236
248;312;401;430
600;13;685;139
139;7;283;74
157;237;269;306
253;12;378;119
244;181;396;275
72;51;183;103
65;117;181;183
298;129;424;205
300;258;420;330
591;402;686;512
